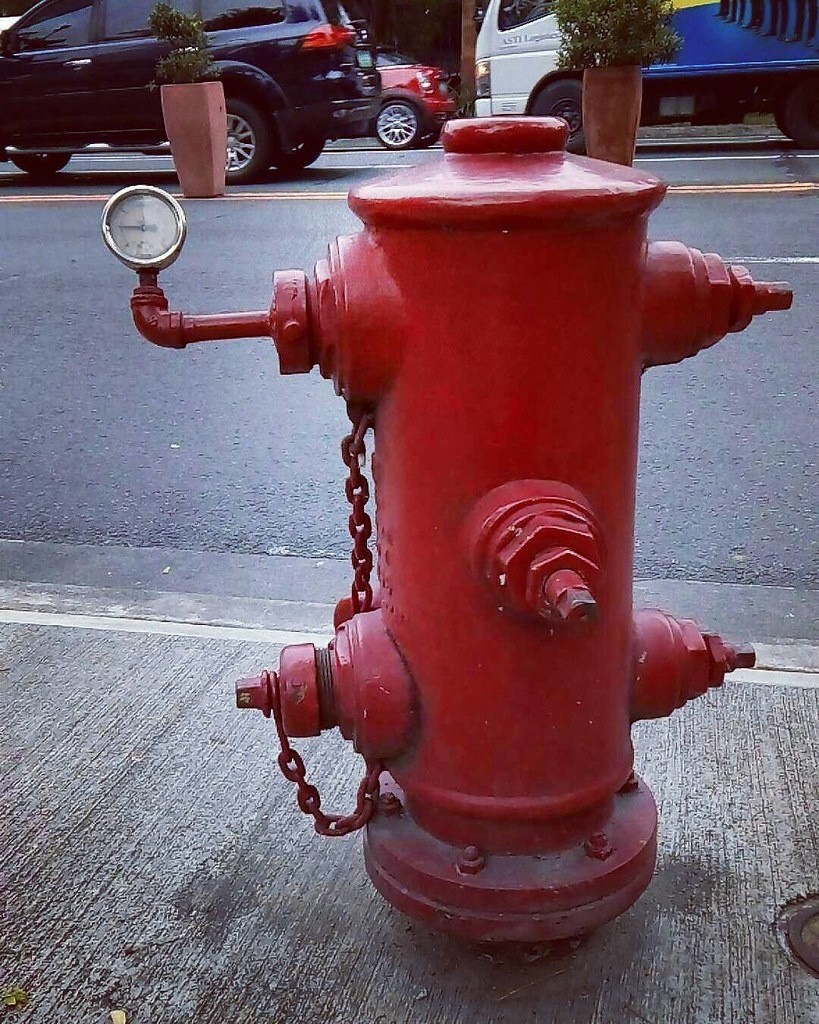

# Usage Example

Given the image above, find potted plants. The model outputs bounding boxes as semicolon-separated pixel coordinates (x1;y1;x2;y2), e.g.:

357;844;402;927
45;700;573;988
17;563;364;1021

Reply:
553;0;684;168
144;3;228;197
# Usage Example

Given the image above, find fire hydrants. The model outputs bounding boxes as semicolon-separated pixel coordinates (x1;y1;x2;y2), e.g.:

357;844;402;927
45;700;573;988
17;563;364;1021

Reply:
99;115;756;942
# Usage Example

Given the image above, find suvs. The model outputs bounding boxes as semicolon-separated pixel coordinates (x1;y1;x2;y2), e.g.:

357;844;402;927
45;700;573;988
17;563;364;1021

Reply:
0;0;383;185
376;43;461;151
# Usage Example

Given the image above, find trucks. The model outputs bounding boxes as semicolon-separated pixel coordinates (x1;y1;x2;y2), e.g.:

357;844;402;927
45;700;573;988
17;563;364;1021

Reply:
474;0;819;155
